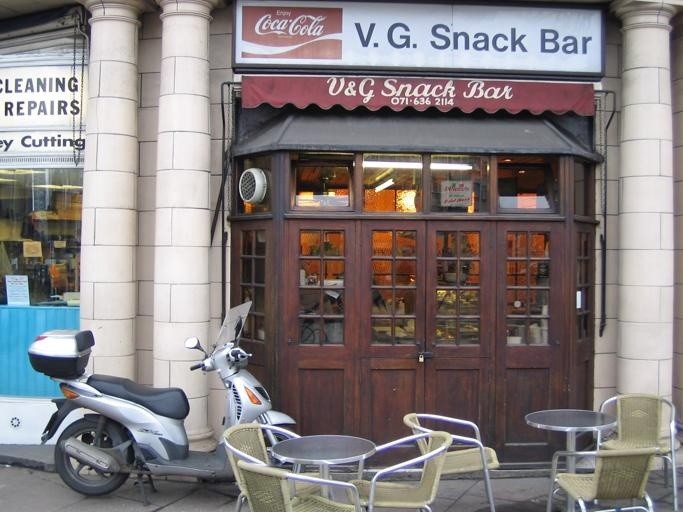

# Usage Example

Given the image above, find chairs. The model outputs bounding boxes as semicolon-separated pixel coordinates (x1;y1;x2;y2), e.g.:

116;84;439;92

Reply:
221;412;500;512
524;393;680;512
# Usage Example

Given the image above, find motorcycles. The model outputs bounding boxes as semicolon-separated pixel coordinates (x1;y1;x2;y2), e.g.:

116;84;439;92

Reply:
25;312;305;508
